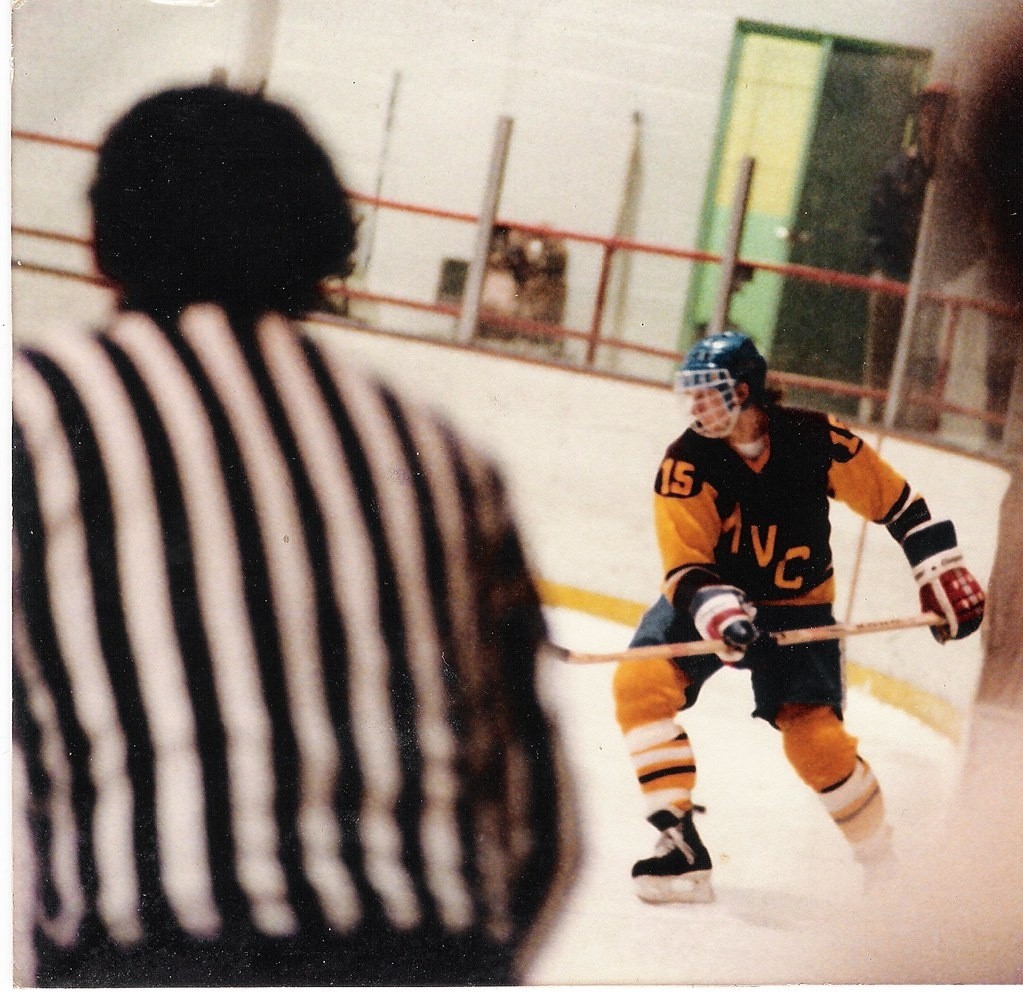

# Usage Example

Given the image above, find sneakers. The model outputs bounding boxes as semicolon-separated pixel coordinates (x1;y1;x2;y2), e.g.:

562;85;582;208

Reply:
630;800;716;906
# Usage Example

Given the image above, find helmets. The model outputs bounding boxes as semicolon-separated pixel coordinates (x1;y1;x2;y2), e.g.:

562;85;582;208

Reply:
676;331;767;440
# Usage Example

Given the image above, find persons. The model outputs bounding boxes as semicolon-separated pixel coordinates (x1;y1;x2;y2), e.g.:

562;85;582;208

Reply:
855;79;981;432
475;224;568;358
12;86;571;988
613;331;985;903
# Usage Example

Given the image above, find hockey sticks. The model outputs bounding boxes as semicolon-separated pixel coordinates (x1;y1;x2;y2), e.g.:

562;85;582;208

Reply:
542;611;947;664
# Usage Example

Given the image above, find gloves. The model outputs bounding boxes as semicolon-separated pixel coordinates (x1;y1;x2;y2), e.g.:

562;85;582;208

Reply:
903;517;986;646
690;585;780;669
